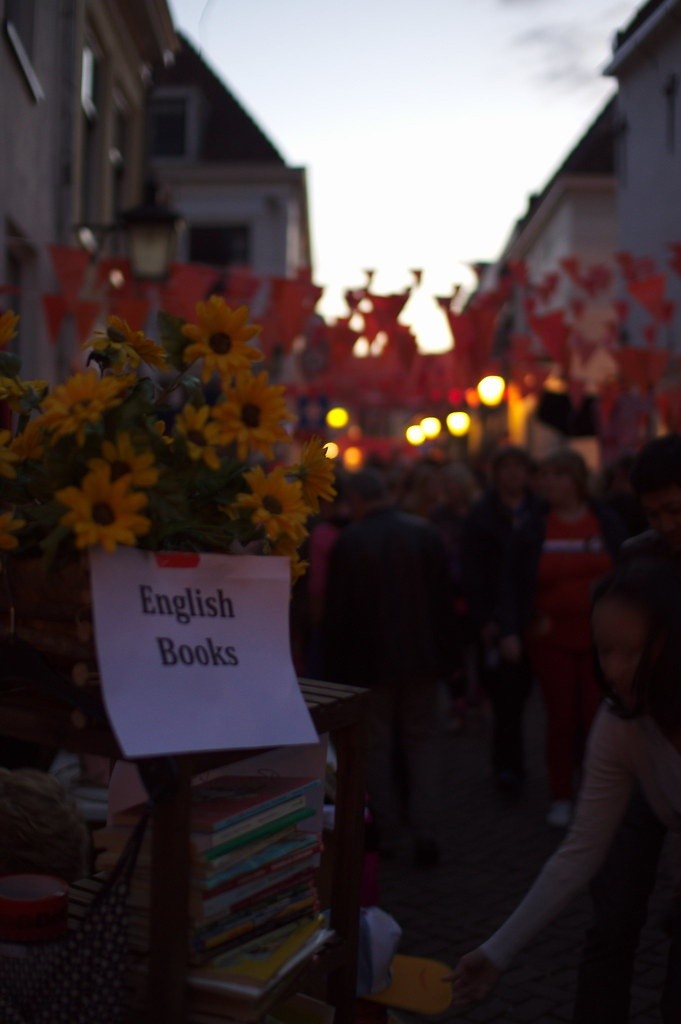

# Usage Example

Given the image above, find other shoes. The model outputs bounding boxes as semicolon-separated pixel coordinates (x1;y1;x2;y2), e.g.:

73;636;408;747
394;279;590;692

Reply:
487;748;505;775
446;701;467;732
396;834;439;867
497;757;528;790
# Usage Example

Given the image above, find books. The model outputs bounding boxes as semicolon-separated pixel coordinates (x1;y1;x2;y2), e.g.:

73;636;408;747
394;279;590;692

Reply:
66;776;336;1024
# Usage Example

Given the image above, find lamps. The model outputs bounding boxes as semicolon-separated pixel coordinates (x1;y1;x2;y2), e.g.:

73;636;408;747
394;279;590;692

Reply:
74;179;186;282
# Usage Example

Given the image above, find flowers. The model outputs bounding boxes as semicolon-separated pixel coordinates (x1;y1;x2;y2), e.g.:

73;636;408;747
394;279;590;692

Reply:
0;297;332;582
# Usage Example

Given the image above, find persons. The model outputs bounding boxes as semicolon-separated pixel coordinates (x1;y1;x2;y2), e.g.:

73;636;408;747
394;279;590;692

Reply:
294;434;681;1023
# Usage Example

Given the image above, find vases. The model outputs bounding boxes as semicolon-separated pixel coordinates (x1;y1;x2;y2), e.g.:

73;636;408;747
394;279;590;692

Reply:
1;560;100;713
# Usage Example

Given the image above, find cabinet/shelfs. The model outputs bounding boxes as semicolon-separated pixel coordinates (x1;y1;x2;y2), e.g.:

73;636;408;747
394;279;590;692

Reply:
0;675;367;1024
